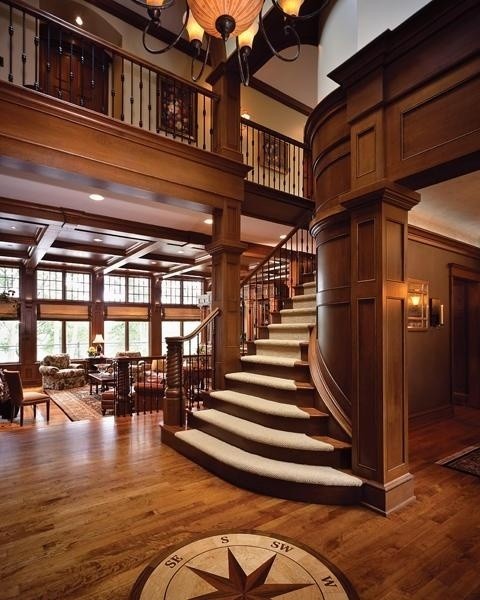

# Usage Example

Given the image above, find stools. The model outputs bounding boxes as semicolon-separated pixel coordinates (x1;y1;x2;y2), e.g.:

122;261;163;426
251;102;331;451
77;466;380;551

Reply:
100;390;115;416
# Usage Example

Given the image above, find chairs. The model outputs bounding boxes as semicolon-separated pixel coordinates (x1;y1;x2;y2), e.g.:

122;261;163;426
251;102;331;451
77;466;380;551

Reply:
113;351;169;384
134;377;167;413
39;354;87;391
2;368;51;428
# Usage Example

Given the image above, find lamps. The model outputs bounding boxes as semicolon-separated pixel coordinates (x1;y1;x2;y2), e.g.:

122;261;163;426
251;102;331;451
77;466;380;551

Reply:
92;333;105;355
133;0;312;89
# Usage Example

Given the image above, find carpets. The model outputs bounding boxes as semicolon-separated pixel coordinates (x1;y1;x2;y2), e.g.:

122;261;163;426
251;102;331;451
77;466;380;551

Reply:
45;387;115;422
434;444;480;480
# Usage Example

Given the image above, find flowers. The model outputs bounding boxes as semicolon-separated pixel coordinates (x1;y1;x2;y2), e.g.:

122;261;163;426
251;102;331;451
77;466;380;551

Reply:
88;347;96;355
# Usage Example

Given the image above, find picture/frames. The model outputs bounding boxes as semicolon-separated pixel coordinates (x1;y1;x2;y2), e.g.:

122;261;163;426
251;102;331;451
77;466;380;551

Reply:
258;131;289;176
154;74;198;141
